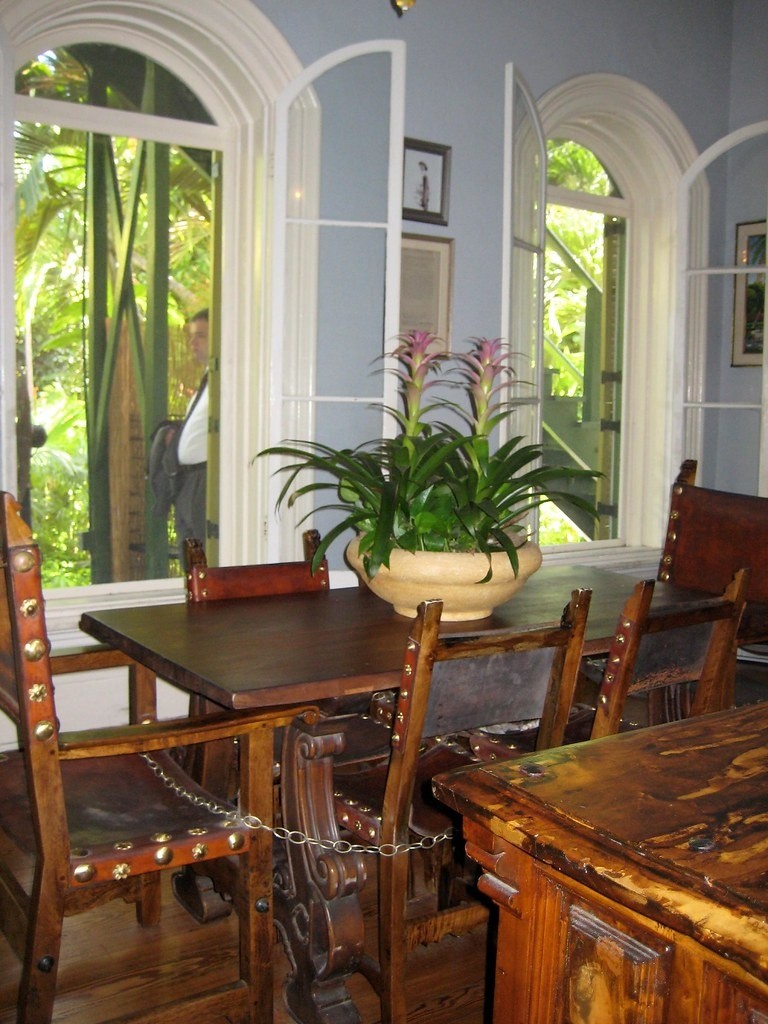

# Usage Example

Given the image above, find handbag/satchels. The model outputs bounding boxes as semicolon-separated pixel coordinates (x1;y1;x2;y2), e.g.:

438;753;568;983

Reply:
161;429;182;475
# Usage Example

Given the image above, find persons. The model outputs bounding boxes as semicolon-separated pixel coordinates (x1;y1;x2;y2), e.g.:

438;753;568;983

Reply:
170;307;215;576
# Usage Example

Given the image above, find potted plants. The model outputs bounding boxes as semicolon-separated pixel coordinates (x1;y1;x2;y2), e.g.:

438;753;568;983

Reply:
261;326;618;618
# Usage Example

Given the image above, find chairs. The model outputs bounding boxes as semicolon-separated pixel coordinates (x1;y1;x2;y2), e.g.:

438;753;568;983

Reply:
0;490;286;1023
584;564;758;749
652;461;768;616
180;522;333;598
324;584;600;1023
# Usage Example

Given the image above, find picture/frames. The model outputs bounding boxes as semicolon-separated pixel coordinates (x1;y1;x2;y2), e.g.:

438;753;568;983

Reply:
396;233;456;360
729;220;766;369
398;134;453;228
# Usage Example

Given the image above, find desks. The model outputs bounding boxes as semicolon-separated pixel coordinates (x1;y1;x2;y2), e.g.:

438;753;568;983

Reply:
76;542;746;1024
